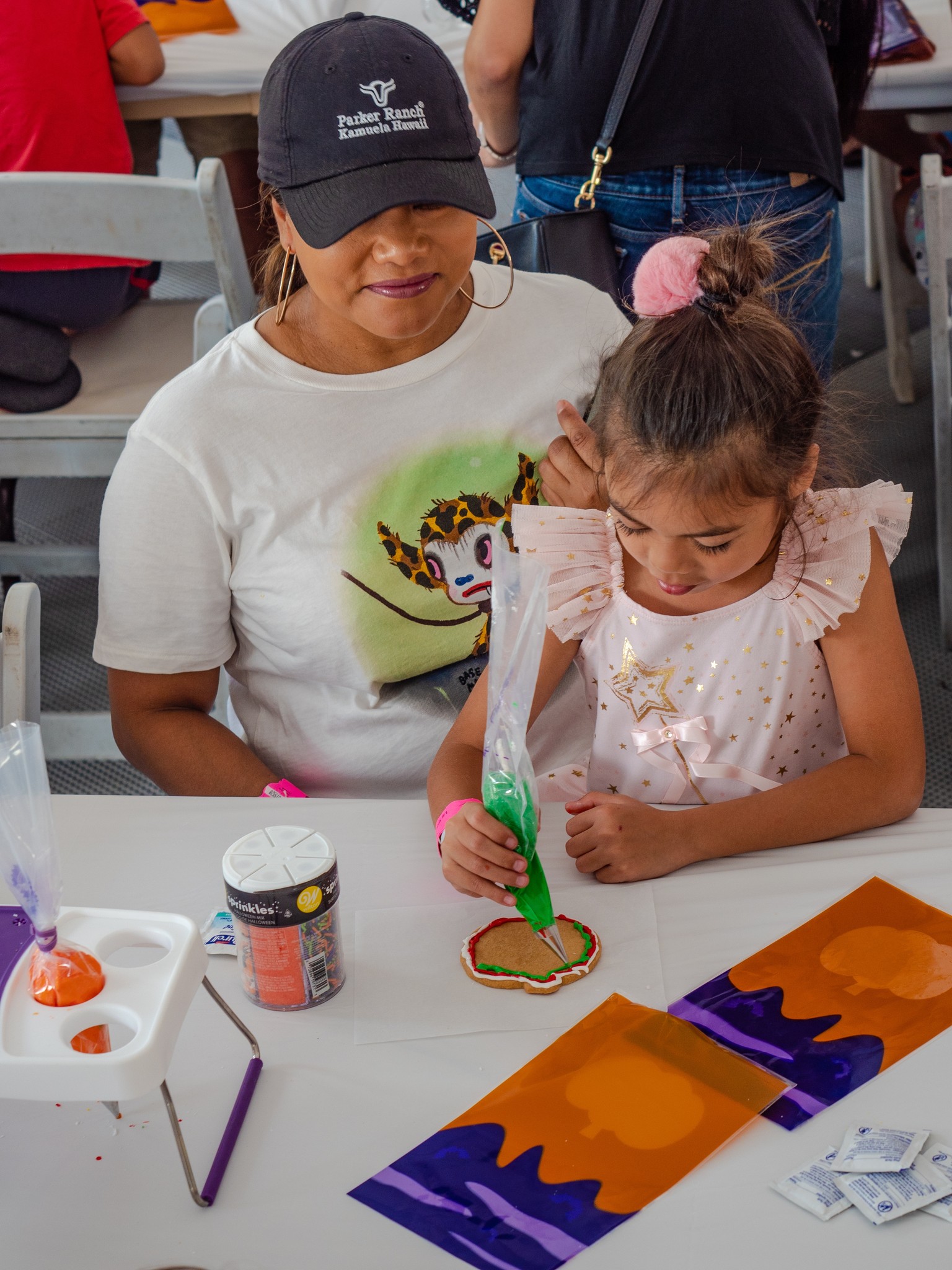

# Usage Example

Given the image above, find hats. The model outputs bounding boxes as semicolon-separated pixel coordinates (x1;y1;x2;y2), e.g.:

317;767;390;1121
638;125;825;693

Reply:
255;10;497;249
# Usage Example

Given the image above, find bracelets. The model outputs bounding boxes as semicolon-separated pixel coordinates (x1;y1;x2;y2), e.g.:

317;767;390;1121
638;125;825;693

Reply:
255;779;308;798
435;798;481;860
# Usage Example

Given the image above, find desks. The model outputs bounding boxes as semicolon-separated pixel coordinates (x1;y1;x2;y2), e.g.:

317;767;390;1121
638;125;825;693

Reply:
2;793;952;1268
853;60;952;403
116;27;496;121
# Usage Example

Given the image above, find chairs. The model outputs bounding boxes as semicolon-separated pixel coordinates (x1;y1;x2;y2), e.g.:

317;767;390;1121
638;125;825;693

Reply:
0;159;271;763
920;153;952;646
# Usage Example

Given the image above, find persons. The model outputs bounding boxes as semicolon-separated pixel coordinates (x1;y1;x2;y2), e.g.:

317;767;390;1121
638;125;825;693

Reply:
464;0;879;385
91;10;635;798
427;190;929;907
0;0;166;332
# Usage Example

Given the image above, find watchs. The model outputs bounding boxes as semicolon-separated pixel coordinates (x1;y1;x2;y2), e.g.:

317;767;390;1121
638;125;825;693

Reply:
477;118;519;161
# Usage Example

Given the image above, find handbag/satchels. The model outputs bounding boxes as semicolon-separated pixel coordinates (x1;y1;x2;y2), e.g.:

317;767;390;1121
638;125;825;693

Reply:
474;205;623;313
